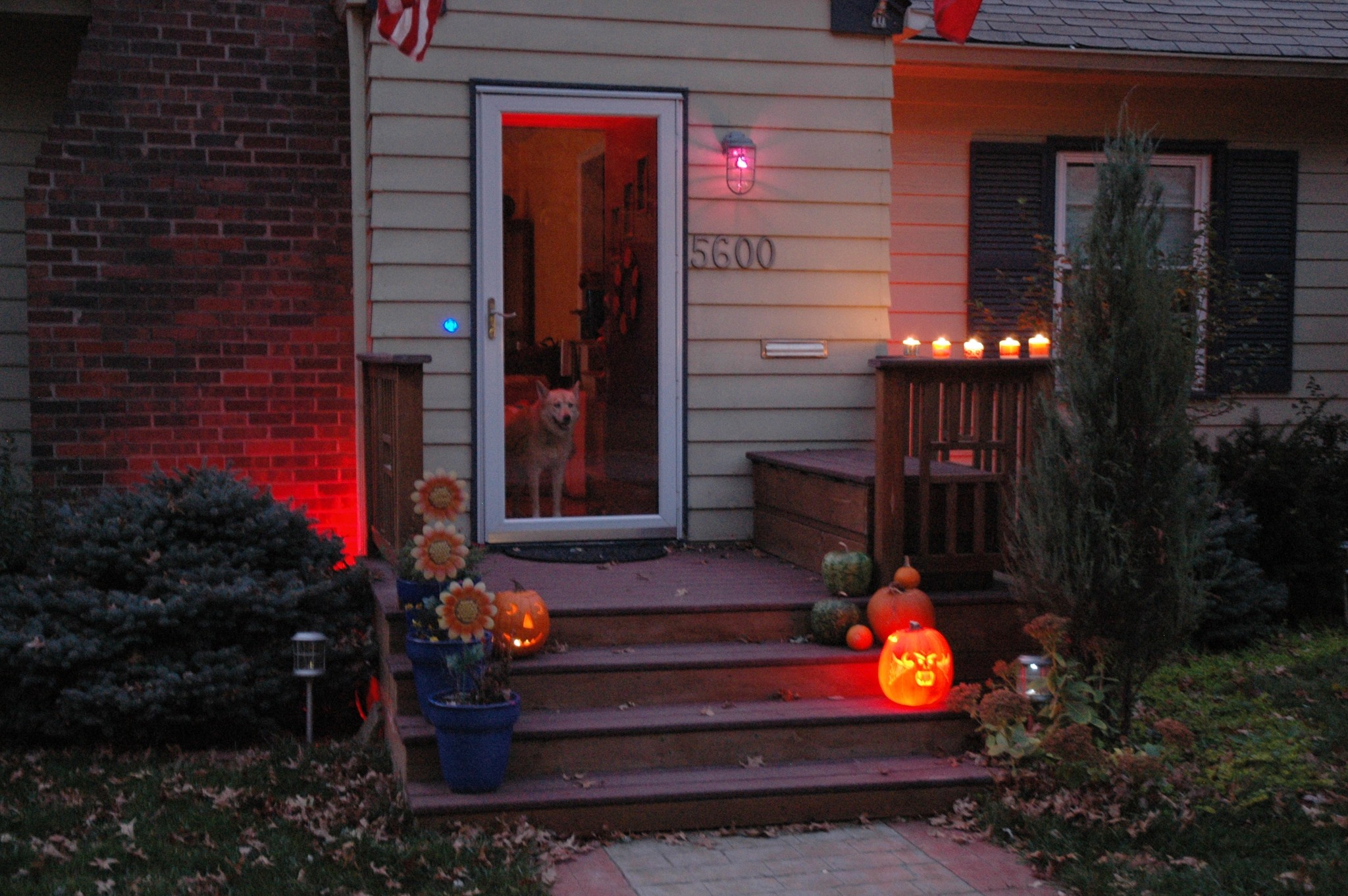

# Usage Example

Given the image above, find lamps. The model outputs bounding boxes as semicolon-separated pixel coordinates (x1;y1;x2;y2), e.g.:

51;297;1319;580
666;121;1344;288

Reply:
760;339;828;358
720;132;756;195
290;632;328;677
1015;655;1054;702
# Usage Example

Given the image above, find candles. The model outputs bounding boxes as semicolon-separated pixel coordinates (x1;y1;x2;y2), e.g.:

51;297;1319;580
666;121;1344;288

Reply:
1029;334;1050;359
903;337;920;359
963;339;984;360
932;338;951;359
1000;337;1020;359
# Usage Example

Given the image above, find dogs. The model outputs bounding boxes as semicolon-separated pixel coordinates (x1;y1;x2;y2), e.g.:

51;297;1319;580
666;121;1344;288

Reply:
504;379;582;518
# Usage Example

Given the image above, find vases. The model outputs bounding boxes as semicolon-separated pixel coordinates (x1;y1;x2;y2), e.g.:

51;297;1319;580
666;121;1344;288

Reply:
425;690;525;794
405;619;494;720
395;575;482;604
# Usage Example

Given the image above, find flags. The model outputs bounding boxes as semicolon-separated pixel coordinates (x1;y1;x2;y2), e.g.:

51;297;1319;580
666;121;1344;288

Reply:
933;0;983;45
376;0;444;63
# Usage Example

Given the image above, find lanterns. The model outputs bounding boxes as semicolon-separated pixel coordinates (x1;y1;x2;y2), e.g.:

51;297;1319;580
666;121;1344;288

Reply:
489;578;550;655
878;621;954;706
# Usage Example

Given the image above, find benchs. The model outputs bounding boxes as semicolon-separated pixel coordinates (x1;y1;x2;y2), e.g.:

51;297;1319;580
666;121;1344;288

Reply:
752;453;1004;589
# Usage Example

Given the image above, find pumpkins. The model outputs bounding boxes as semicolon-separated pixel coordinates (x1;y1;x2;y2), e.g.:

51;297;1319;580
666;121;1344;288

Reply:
821;542;872;596
812;591;859;644
894;555;920;589
847;625;873;650
866;581;935;645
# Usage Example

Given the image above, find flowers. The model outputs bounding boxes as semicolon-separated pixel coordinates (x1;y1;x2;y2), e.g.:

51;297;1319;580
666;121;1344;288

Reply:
400;468;513;700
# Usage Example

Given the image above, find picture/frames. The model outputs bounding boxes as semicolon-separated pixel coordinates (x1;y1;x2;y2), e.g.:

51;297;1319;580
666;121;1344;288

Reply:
622;179;633;239
635;153;649;212
610;205;622;250
